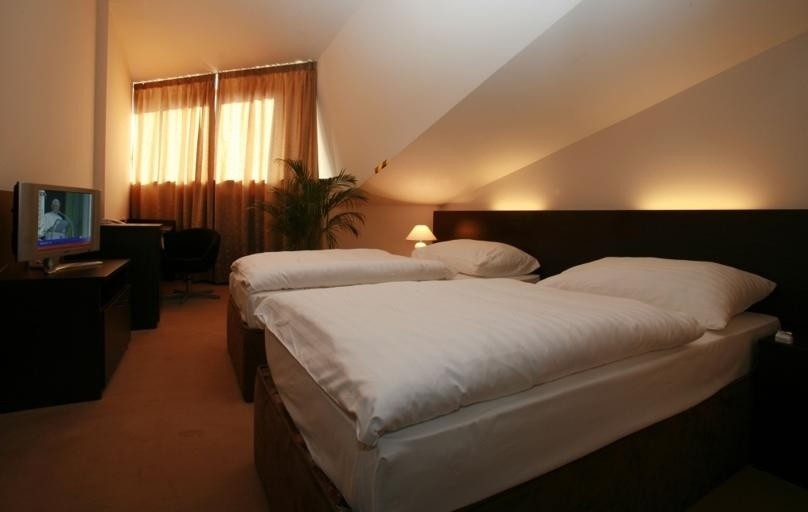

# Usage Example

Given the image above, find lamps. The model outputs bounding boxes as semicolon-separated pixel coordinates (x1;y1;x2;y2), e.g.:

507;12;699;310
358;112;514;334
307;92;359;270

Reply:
403;225;437;248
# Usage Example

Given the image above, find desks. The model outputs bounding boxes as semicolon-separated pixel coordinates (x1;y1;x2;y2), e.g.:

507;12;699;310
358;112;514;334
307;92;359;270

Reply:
64;218;176;329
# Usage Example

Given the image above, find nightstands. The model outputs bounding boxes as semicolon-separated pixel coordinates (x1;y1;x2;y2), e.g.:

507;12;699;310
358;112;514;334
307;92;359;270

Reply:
750;324;808;489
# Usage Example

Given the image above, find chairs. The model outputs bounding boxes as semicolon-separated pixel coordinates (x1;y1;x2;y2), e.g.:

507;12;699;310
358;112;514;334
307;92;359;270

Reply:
169;228;220;304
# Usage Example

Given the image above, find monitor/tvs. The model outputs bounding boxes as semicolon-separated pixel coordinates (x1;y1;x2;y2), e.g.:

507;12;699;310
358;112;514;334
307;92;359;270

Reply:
11;181;103;275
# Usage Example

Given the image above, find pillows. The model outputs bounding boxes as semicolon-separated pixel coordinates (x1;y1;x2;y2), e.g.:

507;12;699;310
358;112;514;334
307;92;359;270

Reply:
411;239;539;278
532;257;777;331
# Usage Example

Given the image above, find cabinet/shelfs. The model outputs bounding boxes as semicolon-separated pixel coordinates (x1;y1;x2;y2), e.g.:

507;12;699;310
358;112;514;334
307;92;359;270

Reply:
0;258;131;414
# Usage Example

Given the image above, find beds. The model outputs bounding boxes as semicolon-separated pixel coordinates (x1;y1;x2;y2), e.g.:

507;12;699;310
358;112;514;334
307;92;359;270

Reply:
226;247;541;403
252;277;794;512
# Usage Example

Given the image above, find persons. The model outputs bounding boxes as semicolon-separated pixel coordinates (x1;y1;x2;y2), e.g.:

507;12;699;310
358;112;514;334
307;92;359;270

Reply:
40;198;66;240
